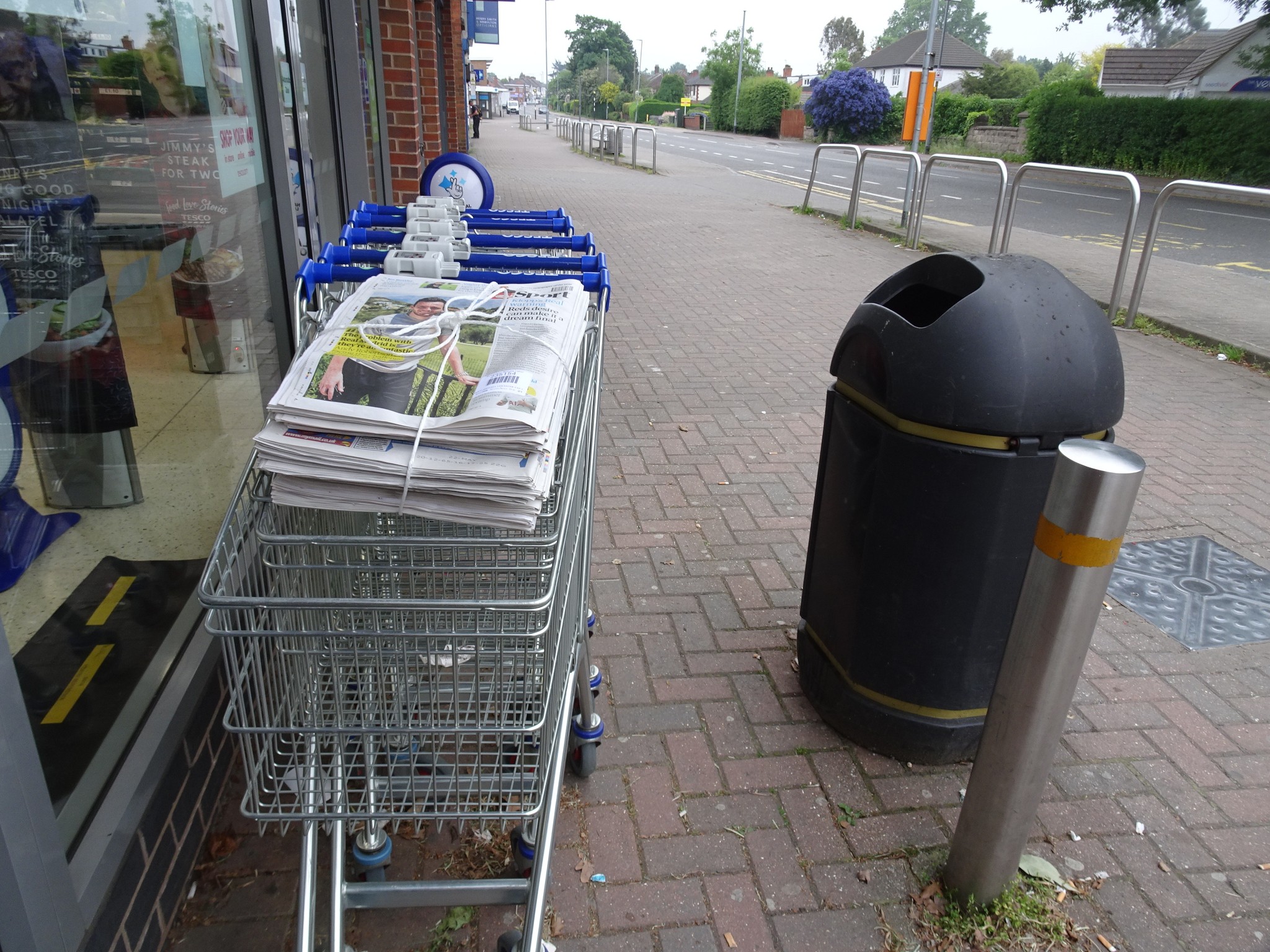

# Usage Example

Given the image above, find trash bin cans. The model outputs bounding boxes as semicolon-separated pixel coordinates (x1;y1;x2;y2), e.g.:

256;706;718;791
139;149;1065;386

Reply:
796;251;1126;766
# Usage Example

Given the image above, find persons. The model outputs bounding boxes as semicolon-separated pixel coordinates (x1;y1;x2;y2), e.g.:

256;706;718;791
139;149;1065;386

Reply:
468;100;480;139
138;17;238;251
318;297;481;414
496;392;538;414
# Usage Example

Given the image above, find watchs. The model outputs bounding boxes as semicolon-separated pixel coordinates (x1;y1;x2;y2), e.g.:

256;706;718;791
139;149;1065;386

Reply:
455;371;469;378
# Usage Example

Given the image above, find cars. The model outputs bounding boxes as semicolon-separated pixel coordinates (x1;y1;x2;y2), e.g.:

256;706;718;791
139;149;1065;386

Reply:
538;105;549;114
533;99;541;104
526;100;535;105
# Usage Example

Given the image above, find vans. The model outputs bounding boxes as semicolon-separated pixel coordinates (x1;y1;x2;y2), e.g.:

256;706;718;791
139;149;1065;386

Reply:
507;100;519;115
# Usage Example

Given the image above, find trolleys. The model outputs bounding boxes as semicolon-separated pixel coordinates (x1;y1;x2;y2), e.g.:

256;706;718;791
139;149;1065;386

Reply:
0;194;101;636
199;201;605;952
